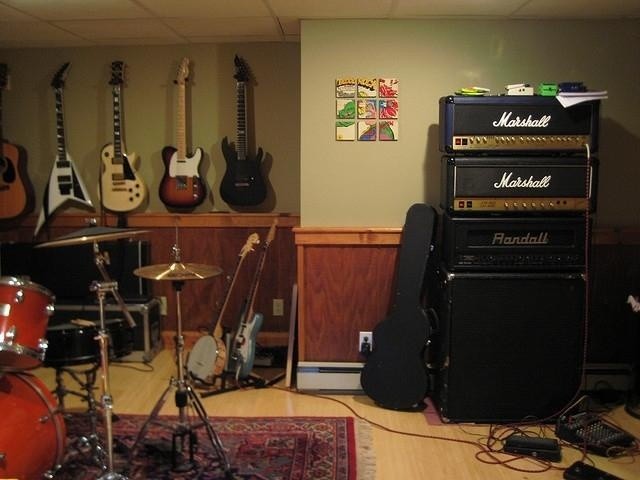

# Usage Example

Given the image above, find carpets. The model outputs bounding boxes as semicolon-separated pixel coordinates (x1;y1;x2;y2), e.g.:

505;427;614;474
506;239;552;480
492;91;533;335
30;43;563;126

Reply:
43;407;373;480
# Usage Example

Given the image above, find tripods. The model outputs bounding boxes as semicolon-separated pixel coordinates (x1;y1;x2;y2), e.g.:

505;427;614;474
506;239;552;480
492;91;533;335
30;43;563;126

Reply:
50;239;137;480
128;285;238;479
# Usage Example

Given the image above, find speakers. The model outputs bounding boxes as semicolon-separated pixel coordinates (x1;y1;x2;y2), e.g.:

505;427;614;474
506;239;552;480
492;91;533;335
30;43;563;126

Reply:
417;270;589;425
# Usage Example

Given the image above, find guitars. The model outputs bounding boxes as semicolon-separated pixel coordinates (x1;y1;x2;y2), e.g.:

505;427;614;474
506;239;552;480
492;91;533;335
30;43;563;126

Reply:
0;63;36;221
96;60;147;213
33;61;95;238
219;54;266;207
223;218;279;380
157;55;207;208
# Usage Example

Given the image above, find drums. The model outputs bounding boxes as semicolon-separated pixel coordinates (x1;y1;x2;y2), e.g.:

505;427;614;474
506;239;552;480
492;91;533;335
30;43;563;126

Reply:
41;318;132;368
0;371;67;479
0;275;56;373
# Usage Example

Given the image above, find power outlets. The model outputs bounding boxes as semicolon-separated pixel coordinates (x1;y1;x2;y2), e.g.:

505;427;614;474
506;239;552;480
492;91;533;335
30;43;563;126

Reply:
359;331;373;355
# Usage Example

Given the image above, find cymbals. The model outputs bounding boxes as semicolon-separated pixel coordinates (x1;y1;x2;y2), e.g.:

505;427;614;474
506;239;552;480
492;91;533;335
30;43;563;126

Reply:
33;227;149;249
133;263;223;280
